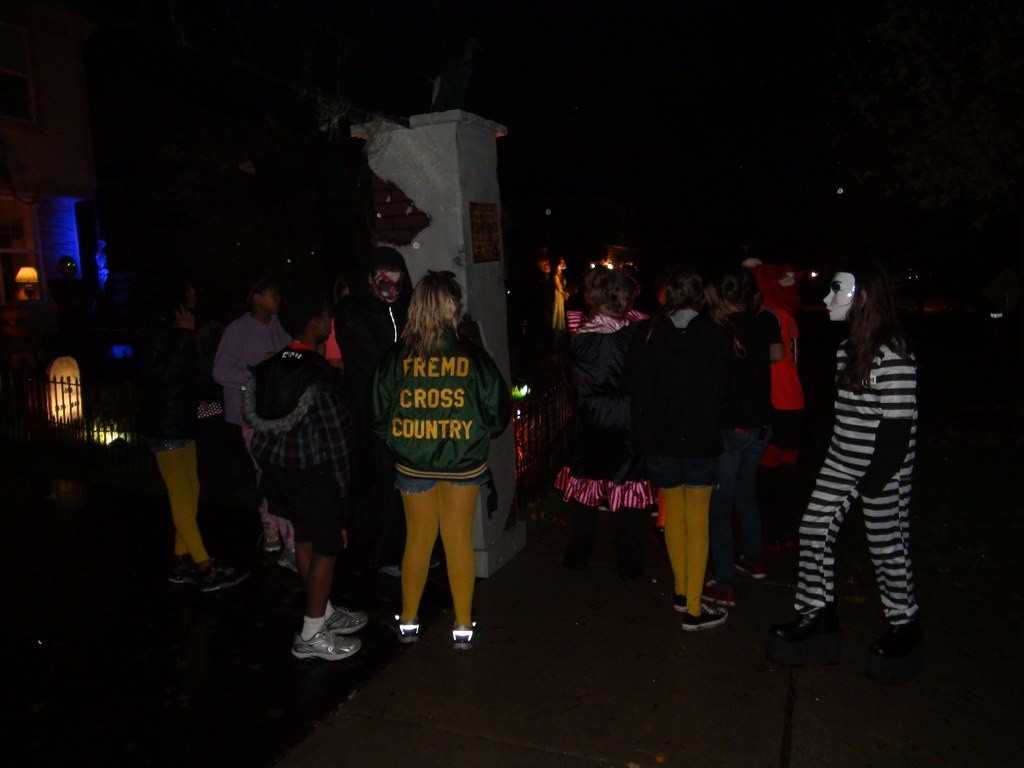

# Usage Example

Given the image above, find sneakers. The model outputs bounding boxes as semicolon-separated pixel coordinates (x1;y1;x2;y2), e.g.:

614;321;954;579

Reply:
197;565;249;592
169;559;201;586
323;607;369;634
700;579;736;607
768;607;840;642
290;627;362;661
734;554;769;579
680;604;729;631
447;615;478;651
669;584;687;611
387;603;419;643
869;622;922;657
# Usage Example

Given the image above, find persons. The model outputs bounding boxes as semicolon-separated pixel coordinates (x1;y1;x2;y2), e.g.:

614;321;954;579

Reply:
767;255;924;660
238;284;370;662
133;262;251;593
620;269;741;632
21;467;128;635
707;263;789;610
211;259;300;579
743;262;808;554
553;259;656;582
366;270;512;650
339;245;417;582
529;244;634;344
